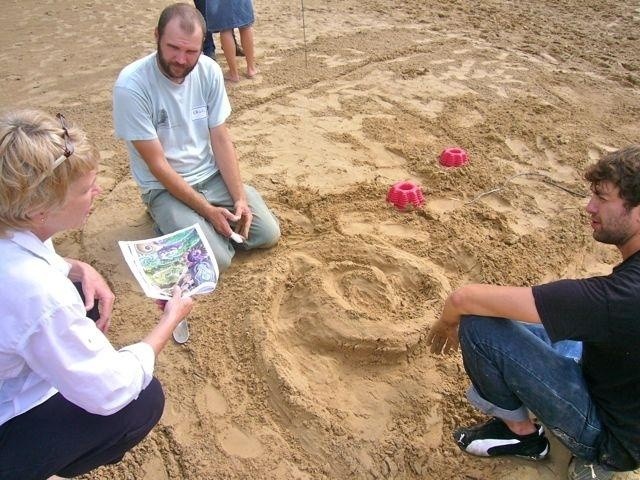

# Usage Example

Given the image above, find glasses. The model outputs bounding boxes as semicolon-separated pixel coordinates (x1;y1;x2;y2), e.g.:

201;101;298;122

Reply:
37;113;74;183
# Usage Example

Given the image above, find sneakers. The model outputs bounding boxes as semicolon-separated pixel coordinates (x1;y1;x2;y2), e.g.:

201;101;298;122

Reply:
204;42;246;61
451;416;551;463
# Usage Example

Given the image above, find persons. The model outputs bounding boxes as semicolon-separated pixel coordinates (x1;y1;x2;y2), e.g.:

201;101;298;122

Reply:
202;2;261;81
425;141;640;479
193;0;245;62
109;0;285;276
1;107;192;480
